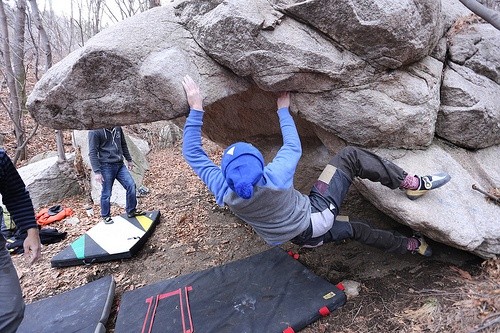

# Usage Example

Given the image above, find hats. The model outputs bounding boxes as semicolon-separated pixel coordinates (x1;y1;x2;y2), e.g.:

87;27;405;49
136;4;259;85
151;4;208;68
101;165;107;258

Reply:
221;141;267;199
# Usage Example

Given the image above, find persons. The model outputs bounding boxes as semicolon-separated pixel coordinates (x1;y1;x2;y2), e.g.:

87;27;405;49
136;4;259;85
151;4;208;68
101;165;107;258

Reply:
86;124;147;226
0;147;43;333
180;74;453;259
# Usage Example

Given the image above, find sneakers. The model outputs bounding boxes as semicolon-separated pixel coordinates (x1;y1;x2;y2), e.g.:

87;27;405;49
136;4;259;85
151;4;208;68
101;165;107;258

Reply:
412;231;432;258
407;173;452;200
127;210;146;218
101;216;113;223
